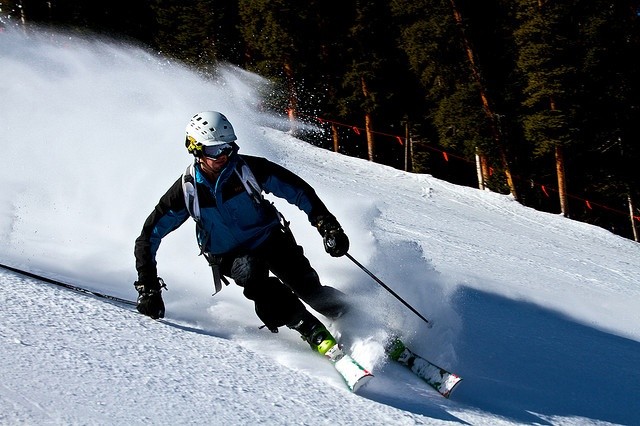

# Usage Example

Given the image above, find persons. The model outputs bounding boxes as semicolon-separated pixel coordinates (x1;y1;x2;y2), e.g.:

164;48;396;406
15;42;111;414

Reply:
133;111;386;357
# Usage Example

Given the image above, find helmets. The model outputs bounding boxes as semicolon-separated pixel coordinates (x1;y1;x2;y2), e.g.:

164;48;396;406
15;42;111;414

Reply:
185;111;237;146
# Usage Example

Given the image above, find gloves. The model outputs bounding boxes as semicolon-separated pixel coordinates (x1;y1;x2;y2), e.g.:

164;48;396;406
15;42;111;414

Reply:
133;281;165;319
317;216;349;257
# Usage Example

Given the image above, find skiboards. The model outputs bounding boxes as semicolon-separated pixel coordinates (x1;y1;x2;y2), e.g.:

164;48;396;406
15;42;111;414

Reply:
307;328;464;399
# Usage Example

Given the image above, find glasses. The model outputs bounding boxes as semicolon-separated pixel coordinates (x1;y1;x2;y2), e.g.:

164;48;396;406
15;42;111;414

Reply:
202;144;239;158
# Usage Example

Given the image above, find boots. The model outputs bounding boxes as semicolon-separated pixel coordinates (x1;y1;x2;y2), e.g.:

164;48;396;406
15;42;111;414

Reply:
286;310;336;356
